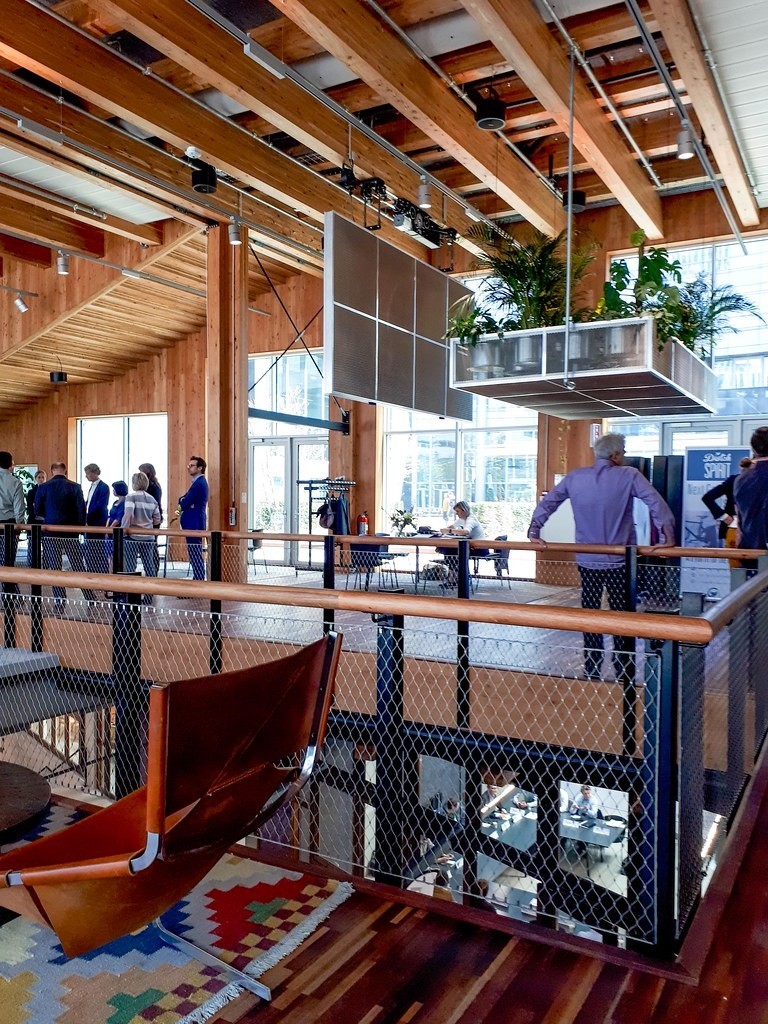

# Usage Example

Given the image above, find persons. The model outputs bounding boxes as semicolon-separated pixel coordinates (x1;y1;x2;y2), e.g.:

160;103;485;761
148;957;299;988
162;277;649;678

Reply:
527;433;675;680
104;480;128;574
702;457;756;570
139;463;163;572
0;451;46;569
437;500;490;589
177;455;208;599
84;463;110;573
34;462;102;614
442;491;455;522
402;785;598;932
733;427;768;593
120;472;160;605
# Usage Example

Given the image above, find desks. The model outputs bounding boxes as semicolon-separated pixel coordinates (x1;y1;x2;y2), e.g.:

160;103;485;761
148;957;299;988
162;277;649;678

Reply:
403;789;625;938
382;533;470;582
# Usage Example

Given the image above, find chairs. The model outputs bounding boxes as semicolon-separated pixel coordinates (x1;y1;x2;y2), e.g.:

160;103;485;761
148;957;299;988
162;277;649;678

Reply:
344;533;414;590
157;535;207;577
0;631;343;1000
605;815;627;843
248;528;268;575
422;534;513;594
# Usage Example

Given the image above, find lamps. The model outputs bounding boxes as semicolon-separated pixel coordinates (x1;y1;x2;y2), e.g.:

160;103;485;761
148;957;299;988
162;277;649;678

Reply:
57;249;69;274
50;356;68;384
228;218;243;245
677;118;694;161
418;177;432;209
191;169;218;194
563;190;585;213
475;99;506;132
14;296;30;313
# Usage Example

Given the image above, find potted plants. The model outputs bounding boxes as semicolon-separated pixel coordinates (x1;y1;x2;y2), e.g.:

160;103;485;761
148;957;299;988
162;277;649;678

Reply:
442;217;766;373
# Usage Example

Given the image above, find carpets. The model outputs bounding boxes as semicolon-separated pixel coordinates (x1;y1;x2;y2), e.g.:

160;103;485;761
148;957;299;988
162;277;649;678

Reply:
0;805;356;1024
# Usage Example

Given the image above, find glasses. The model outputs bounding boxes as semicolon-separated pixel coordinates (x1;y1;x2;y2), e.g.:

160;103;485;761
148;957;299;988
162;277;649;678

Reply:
189;464;198;468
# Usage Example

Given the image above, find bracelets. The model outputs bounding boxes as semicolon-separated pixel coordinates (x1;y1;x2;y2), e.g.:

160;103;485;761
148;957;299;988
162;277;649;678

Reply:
449;529;452;533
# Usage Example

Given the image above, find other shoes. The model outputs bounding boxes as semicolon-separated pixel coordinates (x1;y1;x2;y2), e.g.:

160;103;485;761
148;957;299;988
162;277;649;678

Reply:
439;581;457;589
584;670;600;680
616;674;624;683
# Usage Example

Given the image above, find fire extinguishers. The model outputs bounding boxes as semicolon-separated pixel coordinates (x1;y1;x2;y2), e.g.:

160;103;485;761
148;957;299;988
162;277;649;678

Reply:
357;511;369;537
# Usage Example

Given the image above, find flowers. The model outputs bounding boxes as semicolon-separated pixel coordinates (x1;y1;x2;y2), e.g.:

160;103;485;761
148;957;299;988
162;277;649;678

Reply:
639;304;701;353
